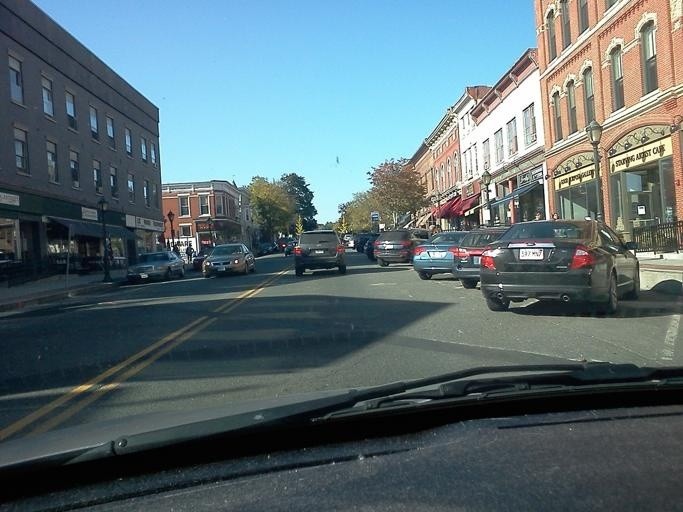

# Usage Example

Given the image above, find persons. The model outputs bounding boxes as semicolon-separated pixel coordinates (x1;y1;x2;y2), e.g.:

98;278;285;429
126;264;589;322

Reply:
552;213;560;220
532;211;543;221
173;245;179;254
186;244;194;265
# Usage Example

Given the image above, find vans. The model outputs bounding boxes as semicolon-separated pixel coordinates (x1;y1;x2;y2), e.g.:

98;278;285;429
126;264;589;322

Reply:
372;230;437;266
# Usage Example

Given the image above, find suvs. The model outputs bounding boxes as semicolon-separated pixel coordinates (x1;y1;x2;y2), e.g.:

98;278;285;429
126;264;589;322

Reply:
293;229;346;277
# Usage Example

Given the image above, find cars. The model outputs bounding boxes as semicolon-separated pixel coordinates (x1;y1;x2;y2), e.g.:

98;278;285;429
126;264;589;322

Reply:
192;244;218;269
342;232;380;261
202;243;256;278
411;231;471;280
451;227;515;289
478;219;642;314
258;237;298;256
126;251;185;283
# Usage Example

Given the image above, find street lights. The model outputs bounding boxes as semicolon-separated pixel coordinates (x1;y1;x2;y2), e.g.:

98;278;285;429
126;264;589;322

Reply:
166;208;175;252
96;197;112;283
205;217;215;246
435;190;442;231
586;119;604;223
481;169;494;226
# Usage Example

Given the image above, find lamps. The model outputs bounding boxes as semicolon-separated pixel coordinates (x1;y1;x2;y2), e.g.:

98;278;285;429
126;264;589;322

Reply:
544;114;682;181
